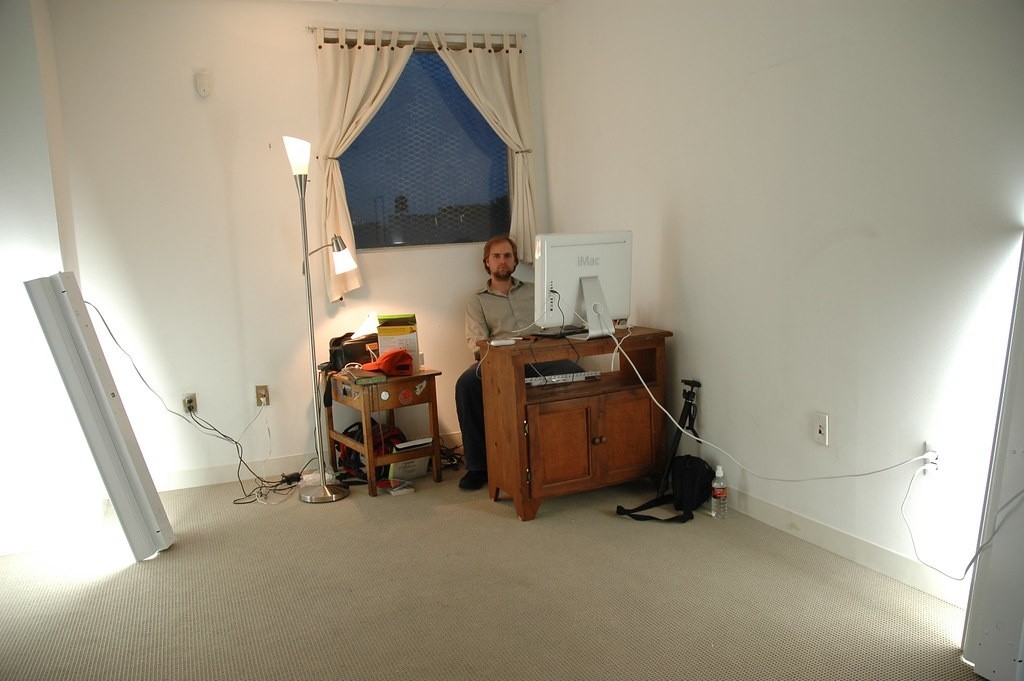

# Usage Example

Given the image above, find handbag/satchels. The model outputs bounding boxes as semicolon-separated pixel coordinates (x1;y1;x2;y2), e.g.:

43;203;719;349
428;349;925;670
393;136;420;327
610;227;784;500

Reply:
616;456;716;522
323;332;379;407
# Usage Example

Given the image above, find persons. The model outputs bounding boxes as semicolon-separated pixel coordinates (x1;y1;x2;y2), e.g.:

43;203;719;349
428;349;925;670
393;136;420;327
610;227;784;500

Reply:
454;234;562;491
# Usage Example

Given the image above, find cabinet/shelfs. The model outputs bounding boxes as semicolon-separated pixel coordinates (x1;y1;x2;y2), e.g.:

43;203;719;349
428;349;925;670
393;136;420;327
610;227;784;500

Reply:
476;324;674;522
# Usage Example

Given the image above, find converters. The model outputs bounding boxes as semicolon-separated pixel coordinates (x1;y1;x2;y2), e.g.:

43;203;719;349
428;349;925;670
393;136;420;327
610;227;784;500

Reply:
285;472;300;485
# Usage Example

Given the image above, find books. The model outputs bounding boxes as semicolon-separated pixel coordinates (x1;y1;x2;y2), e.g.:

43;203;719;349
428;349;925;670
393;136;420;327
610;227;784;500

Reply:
347;370;387;385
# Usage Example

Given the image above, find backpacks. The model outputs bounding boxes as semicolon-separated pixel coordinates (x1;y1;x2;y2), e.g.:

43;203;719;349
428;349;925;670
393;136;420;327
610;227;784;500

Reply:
335;417;407;481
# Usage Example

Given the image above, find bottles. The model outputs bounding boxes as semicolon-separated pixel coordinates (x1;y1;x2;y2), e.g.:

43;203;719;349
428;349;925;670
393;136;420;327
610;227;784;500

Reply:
712;465;728;520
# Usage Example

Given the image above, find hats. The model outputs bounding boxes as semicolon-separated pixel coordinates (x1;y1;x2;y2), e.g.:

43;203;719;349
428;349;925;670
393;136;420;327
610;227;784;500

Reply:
362;349;413;376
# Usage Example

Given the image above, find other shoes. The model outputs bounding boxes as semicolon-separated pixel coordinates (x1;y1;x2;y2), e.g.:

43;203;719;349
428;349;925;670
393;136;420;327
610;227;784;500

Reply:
458;471;488;490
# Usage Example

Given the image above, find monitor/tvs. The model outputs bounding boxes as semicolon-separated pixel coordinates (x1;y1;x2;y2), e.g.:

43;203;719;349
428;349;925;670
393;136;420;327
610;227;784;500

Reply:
534;229;633;341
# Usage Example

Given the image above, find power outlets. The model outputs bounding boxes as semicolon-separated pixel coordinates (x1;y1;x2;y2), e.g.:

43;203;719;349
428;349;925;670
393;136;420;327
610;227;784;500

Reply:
813;410;829;446
923;440;953;477
180;392;198;414
254;385;271;408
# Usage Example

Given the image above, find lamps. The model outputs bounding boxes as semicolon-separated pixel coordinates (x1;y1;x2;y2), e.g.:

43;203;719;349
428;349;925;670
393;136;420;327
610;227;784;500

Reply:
283;134;357;504
961;233;1024;681
25;271;177;562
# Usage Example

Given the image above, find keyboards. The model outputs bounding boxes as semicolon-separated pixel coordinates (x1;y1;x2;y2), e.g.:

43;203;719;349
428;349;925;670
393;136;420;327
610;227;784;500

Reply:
530;328;589;338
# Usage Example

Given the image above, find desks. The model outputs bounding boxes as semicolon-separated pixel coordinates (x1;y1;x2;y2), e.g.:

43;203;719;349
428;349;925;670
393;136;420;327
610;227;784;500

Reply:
321;364;442;496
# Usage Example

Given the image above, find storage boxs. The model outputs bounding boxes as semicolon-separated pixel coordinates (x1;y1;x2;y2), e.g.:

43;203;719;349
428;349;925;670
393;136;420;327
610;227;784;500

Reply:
376;313;424;374
388;437;434;481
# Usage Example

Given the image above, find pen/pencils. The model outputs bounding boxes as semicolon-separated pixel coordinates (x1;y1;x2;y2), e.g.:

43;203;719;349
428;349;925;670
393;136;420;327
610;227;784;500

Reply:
505;337;530;341
